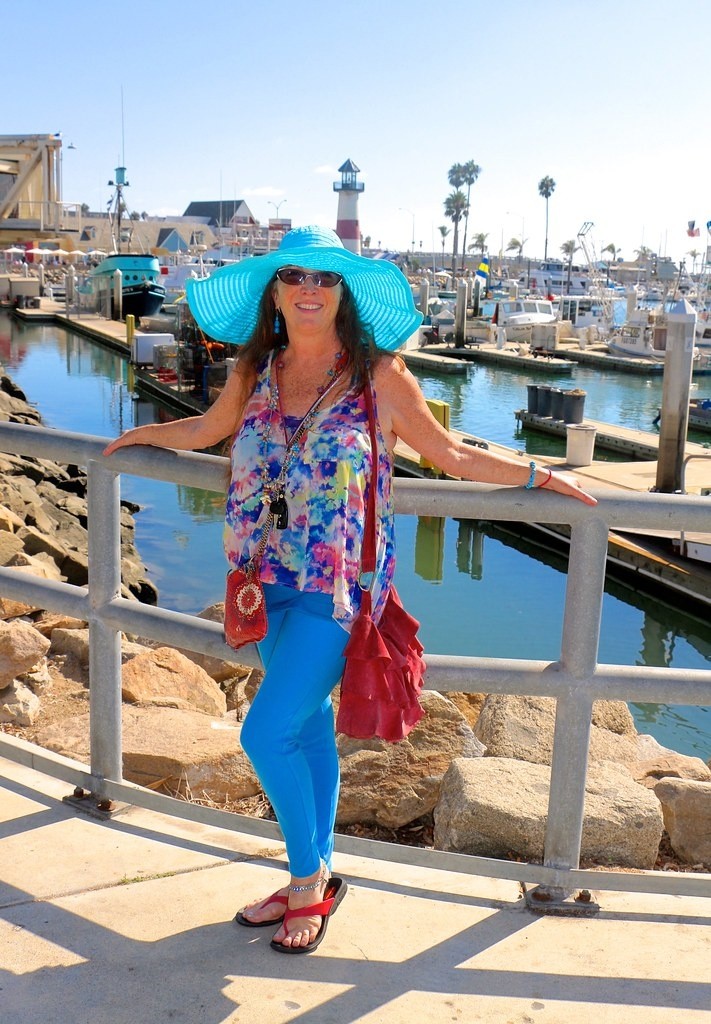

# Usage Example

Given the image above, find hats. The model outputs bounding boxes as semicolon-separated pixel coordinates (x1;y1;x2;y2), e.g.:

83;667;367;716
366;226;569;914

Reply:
184;225;424;352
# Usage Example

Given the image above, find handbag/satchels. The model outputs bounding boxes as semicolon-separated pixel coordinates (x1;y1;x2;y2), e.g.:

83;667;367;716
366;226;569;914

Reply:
336;583;428;741
226;555;268;649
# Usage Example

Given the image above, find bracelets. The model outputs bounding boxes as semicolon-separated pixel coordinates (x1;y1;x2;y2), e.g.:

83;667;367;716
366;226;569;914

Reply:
525;461;537;490
538;468;551;487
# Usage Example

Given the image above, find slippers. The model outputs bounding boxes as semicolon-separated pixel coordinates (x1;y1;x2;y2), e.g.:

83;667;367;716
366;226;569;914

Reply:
271;877;347;954
236;884;290;927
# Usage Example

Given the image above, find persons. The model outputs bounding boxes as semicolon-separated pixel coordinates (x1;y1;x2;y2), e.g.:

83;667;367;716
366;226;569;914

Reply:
102;224;597;955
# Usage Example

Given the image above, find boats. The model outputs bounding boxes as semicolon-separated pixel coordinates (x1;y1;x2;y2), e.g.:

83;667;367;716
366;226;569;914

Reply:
71;81;167;322
418;221;711;440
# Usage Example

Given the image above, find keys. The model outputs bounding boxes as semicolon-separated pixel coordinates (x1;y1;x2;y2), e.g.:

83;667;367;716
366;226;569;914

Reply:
267;497;288;534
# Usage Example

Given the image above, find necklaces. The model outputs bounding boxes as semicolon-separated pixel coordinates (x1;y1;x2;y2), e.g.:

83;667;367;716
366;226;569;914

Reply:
259;342;352;507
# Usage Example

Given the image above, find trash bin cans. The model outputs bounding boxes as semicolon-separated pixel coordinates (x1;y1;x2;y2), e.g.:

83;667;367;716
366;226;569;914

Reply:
527;384;546;414
563;392;586;423
550;389;571;419
565;423;597;466
537;386;560;416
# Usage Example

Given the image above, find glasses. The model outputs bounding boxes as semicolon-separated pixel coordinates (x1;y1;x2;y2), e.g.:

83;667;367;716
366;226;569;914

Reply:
276;269;343;288
